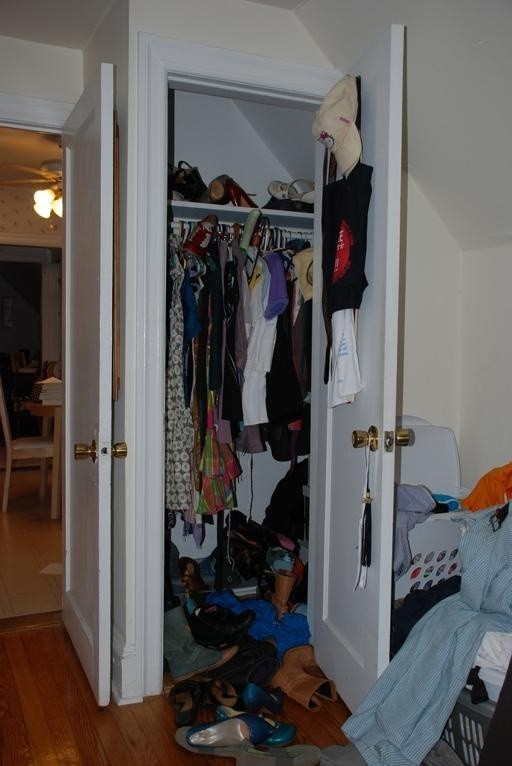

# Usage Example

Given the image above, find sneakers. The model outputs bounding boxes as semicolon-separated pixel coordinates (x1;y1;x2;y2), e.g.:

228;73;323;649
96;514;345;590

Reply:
236;745;323;765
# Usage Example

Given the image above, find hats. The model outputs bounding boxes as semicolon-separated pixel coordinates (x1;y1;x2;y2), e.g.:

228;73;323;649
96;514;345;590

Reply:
312;74;362;176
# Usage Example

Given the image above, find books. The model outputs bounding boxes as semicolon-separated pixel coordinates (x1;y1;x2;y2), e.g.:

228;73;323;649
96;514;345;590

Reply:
31;377;65;404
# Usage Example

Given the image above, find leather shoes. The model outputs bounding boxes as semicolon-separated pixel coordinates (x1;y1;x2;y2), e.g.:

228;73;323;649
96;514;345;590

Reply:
183;213;218;257
165;606;295;756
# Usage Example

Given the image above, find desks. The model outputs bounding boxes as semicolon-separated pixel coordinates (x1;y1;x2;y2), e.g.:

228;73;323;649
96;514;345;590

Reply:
23;398;62;521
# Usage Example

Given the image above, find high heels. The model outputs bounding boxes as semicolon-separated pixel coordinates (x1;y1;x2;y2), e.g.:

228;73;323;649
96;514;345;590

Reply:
201;174;259;208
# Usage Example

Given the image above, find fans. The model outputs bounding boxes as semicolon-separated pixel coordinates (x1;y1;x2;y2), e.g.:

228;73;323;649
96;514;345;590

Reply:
0;159;63;189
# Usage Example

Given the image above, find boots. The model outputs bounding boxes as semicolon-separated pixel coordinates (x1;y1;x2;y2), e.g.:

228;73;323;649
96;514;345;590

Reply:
271;645;337;712
265;571;296;623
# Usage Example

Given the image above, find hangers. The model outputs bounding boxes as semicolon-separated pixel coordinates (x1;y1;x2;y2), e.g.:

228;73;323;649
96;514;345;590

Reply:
172;221;314;269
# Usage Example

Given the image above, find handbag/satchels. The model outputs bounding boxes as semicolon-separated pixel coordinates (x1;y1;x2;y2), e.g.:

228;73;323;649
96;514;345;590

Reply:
168;161;207;202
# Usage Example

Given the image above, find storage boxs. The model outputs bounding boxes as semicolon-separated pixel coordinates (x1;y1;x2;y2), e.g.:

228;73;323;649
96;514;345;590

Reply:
392;485;480;601
415;646;512;766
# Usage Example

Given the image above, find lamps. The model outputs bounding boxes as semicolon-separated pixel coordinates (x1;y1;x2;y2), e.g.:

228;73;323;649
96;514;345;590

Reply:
32;179;64;219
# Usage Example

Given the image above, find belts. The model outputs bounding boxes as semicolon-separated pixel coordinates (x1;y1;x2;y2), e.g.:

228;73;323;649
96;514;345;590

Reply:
489;502;510;531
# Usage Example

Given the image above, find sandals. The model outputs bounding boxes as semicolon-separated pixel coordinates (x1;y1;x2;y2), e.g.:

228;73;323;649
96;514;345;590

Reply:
268;178;314;205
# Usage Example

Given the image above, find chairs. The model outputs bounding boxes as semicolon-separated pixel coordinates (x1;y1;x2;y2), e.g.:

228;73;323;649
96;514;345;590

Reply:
0;380;57;512
